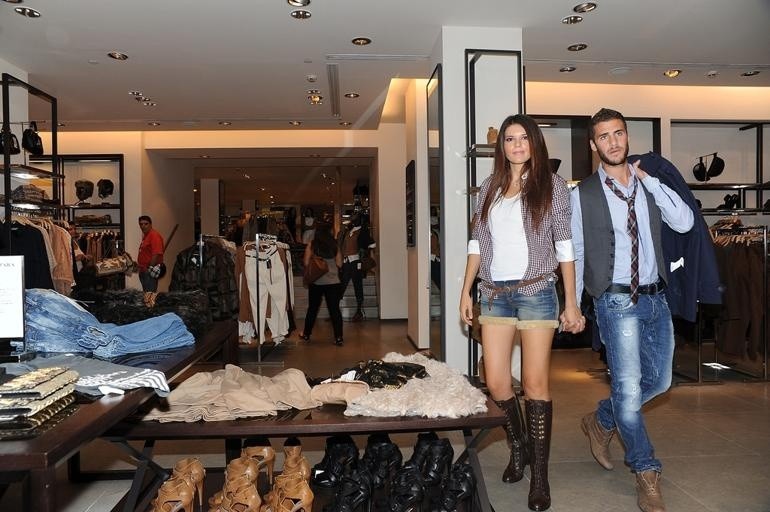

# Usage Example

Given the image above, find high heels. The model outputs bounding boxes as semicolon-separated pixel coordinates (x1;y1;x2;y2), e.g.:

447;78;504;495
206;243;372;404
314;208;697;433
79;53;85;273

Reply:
207;431;476;512
150;457;207;512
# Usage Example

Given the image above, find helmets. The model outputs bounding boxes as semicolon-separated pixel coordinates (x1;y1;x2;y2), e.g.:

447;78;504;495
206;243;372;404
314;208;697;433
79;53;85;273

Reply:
148;263;166;279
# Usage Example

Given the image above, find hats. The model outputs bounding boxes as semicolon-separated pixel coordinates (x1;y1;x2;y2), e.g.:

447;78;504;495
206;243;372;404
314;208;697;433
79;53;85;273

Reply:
75;180;94;197
97;179;114;195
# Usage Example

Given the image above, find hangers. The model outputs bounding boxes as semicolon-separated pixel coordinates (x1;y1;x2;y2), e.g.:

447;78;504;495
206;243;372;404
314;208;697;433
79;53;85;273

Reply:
199;233;286;249
711;215;769;247
0;208;54;229
74;225;122;241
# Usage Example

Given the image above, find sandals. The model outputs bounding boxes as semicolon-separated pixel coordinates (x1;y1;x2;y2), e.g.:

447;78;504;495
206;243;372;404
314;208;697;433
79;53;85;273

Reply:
299;333;310;343
333;337;344;345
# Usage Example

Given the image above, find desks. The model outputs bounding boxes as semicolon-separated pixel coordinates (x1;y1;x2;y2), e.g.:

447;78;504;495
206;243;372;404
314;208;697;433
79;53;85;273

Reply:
0;316;242;511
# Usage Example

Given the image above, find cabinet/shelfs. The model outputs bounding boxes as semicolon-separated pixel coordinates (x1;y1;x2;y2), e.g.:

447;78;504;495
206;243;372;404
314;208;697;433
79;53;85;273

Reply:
29;152;125;290
101;374;510;511
0;72;65;255
300;203;336;242
669;118;769;216
270;206;297;238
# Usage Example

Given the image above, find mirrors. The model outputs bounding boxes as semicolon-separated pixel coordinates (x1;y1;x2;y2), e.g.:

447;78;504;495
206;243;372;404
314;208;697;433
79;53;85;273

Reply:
465;48;522;396
426;63;445;363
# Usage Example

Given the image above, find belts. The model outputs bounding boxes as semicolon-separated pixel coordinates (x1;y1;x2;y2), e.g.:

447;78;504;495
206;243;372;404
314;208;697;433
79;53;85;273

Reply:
606;280;665;295
481;276;544;311
242;248;277;262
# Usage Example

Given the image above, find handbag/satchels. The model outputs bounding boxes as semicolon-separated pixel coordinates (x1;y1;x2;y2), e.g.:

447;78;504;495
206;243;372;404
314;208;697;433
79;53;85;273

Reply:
303;256;328;285
1;127;20;155
23;128;44;157
0;367;79;432
97;253;134;277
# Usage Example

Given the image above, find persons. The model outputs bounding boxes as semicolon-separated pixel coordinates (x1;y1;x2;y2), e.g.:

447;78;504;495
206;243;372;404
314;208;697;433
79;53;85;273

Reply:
298;221;343;345
325;223;377;322
460;115;583;512
558;108;694;512
138;216;164;292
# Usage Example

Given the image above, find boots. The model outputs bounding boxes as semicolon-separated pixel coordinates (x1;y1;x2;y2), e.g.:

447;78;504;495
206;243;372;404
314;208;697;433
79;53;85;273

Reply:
492;392;531;484
637;470;665;512
581;411;617;471
524;399;552;512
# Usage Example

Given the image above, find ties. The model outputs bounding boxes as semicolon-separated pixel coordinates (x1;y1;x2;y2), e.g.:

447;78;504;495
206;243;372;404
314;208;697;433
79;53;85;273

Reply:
605;176;638;304
